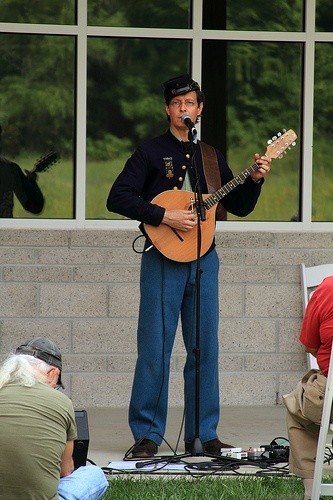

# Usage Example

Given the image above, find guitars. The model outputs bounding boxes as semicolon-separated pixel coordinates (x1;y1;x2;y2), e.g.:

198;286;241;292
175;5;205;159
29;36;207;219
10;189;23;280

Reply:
27;151;61;177
144;128;298;262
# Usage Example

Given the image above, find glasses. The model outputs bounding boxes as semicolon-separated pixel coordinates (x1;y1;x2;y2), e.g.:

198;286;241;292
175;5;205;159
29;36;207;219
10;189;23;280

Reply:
170;100;197;106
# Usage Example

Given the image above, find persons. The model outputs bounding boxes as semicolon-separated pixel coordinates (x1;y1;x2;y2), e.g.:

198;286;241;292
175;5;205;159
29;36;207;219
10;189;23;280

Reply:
107;75;272;458
282;276;333;500
0;125;45;218
0;338;109;500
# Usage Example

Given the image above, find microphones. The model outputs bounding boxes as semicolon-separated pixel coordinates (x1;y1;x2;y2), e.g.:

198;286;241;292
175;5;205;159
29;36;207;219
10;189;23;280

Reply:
182;114;197;135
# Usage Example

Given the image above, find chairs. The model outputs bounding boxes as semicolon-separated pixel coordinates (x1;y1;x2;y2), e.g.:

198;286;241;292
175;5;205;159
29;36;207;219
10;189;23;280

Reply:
311;346;333;500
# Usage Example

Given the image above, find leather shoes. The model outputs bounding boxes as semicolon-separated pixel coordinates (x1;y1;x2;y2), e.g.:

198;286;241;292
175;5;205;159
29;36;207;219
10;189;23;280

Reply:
185;440;233;459
133;438;156;459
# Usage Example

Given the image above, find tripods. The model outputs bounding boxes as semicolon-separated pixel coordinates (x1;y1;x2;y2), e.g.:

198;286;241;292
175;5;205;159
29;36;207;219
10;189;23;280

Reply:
135;133;273;468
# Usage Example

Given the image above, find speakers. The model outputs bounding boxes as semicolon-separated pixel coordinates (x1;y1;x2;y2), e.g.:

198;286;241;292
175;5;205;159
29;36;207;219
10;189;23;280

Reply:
70;410;90;471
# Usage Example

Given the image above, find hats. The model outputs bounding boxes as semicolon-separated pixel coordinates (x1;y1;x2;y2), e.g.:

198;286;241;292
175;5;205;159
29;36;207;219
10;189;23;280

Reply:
15;337;65;389
163;75;200;105
